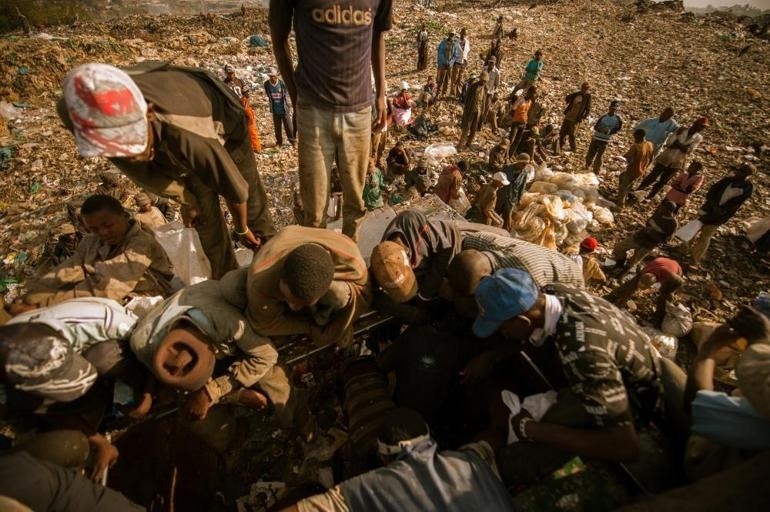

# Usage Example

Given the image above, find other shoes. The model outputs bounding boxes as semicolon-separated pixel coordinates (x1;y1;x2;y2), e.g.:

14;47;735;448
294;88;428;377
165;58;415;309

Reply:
672;248;687;256
615;269;629;279
689;265;698;274
613;260;625;269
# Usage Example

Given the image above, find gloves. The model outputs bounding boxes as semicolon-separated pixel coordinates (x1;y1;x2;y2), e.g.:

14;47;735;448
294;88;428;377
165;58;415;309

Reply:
511;408;536;443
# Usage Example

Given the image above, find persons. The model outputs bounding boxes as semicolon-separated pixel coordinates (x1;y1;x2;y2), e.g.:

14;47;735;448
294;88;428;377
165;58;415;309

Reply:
1;0;770;512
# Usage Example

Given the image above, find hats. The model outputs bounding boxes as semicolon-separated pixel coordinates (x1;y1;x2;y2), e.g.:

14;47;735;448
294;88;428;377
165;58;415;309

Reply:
134;189;158;207
395;28;710;215
368;421;430;456
267;67;277;76
65;62;148;158
472;267;538;338
152;328;216;395
741;161;757;176
581;237;597;251
735;342;770;418
224;63;235;73
0;322;98;403
103;173;114;189
638;272;658;289
370;240;418;303
